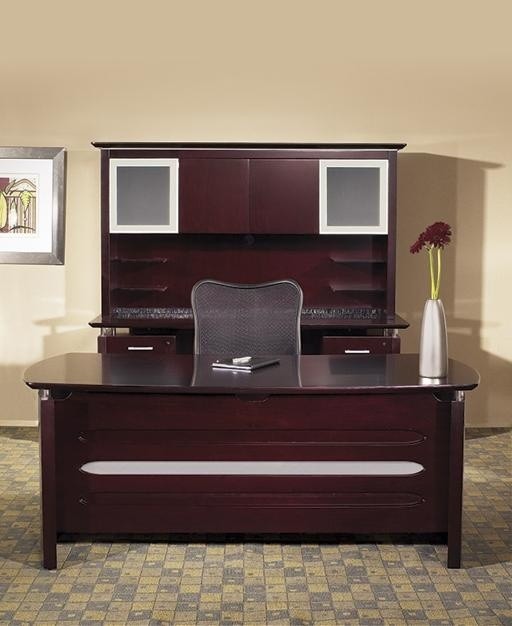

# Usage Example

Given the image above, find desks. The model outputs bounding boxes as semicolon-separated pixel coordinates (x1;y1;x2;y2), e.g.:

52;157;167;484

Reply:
21;350;482;573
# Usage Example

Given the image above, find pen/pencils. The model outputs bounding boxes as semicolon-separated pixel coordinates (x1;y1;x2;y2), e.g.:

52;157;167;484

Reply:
230;355;253;363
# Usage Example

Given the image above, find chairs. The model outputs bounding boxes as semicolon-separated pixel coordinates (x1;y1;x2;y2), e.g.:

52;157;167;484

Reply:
192;357;305;393
188;276;306;360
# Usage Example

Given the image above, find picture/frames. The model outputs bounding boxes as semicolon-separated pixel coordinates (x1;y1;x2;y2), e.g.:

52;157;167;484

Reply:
0;141;70;267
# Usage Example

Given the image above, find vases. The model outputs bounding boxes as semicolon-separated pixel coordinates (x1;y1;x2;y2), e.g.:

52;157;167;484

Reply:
416;297;452;381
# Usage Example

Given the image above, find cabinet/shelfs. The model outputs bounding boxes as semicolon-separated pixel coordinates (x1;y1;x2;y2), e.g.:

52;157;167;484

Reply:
241;140;409;237
89;140;249;237
85;308;414;356
99;233;399;320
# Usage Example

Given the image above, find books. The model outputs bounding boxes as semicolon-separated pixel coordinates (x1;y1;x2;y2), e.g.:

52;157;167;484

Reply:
211;355;281;370
212;366;275;374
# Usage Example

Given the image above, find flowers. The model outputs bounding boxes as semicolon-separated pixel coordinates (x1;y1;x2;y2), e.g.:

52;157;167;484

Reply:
408;213;455;303
